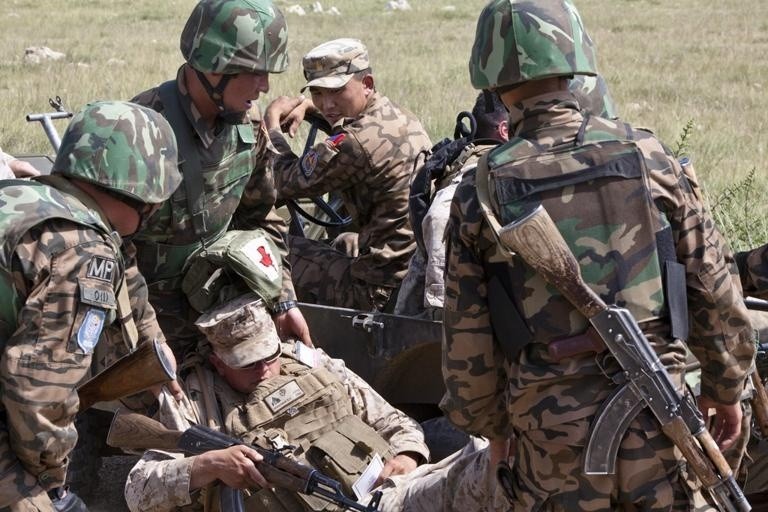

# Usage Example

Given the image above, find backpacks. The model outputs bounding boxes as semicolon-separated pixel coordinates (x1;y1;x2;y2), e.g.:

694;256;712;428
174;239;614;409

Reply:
483;130;671;366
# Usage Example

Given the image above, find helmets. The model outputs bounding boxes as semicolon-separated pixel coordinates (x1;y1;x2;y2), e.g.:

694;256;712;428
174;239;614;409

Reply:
181;0;289;74
468;0;618;121
51;102;183;204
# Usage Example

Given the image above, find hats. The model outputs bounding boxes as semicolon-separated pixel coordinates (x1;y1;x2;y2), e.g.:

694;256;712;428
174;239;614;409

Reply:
196;290;279;369
301;37;368;93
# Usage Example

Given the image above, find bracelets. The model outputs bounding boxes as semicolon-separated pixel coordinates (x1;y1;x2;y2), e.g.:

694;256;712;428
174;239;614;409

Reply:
271;302;299;314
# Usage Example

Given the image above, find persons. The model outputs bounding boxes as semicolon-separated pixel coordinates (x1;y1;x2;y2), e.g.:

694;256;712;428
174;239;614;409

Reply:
438;0;767;511
125;294;511;512
129;2;314;351
1;100;183;512
421;90;512;310
262;38;435;315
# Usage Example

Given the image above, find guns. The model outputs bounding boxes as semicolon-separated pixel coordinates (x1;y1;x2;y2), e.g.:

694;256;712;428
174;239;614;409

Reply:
75;340;176;412
108;407;377;511
498;203;753;512
